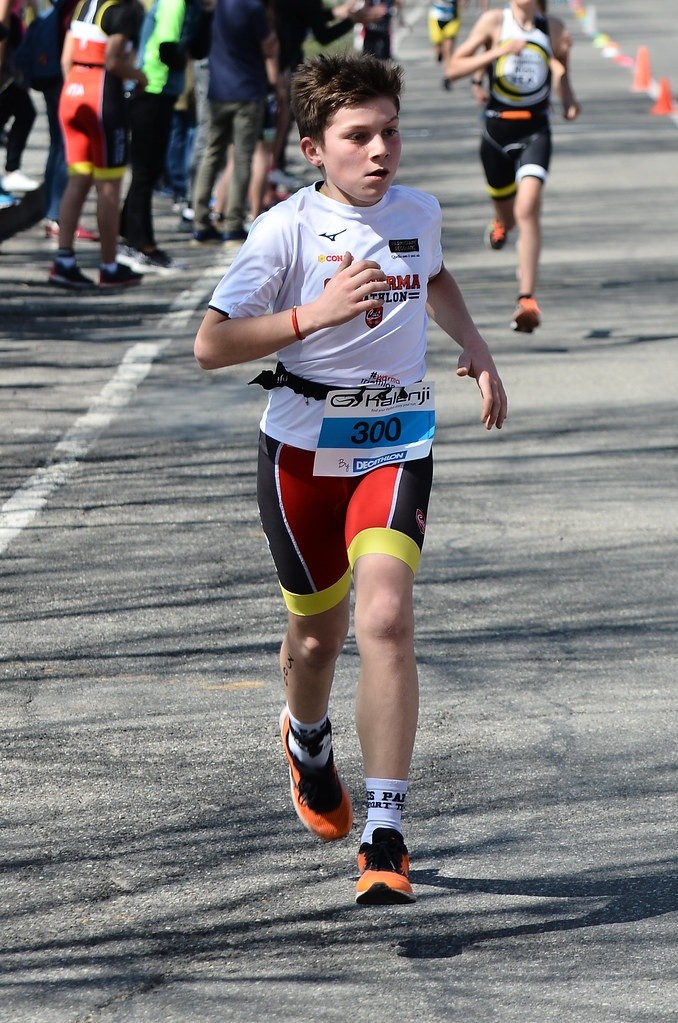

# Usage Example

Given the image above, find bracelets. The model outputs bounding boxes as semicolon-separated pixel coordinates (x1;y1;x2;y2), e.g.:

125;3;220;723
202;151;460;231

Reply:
292;305;304;340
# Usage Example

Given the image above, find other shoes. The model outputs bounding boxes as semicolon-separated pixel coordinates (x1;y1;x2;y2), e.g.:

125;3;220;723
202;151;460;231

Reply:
188;225;226;248
243;215;256;233
178;218;194;232
46;219;96;240
0;170;40;193
119;212;171;267
222;228;249;251
0;194;14;209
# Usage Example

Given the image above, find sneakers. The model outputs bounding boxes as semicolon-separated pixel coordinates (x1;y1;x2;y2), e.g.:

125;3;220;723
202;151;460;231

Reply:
99;263;144;290
509;301;539;333
49;261;96;290
277;708;352;838
485;216;509;249
356;826;414;905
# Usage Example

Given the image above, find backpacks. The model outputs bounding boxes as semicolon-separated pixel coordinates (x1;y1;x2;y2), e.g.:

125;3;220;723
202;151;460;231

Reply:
14;8;64;90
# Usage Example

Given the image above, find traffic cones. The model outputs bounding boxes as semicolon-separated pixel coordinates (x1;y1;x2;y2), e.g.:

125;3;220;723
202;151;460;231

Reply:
631;45;656;95
650;77;678;116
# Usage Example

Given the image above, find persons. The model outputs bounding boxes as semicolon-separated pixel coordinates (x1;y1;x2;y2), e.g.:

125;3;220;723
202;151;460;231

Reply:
0;0;414;290
444;0;581;334
194;57;508;905
426;0;470;90
